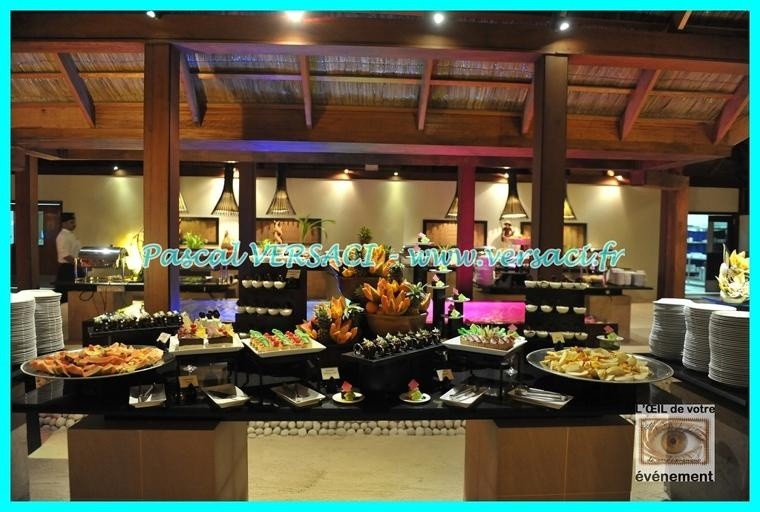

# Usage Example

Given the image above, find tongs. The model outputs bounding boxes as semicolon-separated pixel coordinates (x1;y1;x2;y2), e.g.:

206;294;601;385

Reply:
137;380;157;403
515;384;568;402
282;381;303;402
448;383;480;401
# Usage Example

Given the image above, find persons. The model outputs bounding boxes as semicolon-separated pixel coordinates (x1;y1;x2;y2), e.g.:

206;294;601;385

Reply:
54;210;82;278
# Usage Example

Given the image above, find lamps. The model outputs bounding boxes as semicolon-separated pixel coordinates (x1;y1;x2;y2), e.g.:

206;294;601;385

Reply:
264;164;299;216
212;162;239;218
443;181;459;219
498;165;528;220
564;173;578;221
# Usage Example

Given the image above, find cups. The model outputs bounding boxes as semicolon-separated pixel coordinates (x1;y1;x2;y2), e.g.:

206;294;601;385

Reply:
608;267;646;286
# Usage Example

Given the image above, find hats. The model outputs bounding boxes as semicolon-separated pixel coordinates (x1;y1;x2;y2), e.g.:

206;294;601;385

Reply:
60;212;75;222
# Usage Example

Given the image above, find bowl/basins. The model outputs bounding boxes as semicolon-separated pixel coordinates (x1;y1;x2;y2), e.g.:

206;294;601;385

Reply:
573;307;587;314
556;305;570;314
236;305;294;317
526;304;538;314
540;305;553;313
524;329;588;341
523;280;589;291
242;279;287;290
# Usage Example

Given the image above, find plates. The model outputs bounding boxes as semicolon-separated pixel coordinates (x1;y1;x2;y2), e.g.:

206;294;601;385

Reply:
439;383;491;409
506;387;574;410
200;382;251;410
525;345;675;386
596;335;624;341
19;344;177;381
271;383;326;409
399;391;432;403
128;383;167;409
332;391;366;404
441;313;463;320
412;241;435;246
447;296;471;303
647;296;749;388
11;288;65;366
427;285;450;289
429;269;453;274
